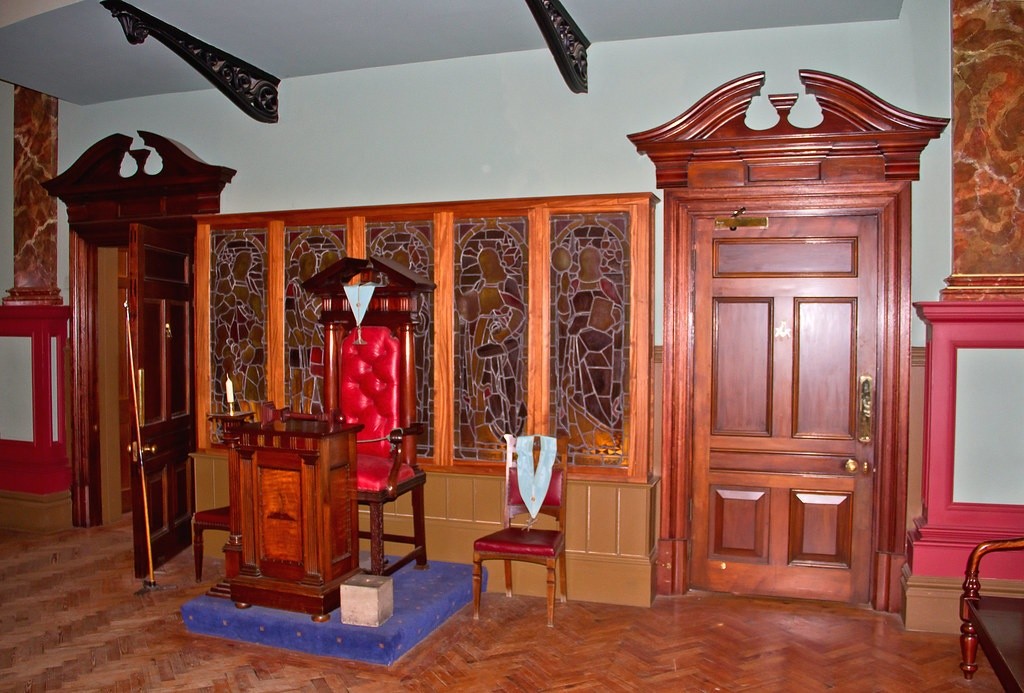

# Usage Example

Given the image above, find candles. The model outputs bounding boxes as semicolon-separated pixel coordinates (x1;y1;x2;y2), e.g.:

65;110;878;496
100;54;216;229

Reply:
226;373;234;403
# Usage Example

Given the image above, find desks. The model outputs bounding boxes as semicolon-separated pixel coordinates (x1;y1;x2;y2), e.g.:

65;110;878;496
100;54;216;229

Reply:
219;420;364;622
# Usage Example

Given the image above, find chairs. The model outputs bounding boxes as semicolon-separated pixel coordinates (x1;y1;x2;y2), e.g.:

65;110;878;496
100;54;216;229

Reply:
473;434;569;627
301;256;437;576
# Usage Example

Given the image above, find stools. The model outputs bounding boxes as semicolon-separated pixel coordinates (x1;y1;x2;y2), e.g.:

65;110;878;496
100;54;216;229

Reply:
194;506;230;583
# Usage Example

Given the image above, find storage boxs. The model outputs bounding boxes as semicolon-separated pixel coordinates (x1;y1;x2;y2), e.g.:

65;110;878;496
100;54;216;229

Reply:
340;573;393;627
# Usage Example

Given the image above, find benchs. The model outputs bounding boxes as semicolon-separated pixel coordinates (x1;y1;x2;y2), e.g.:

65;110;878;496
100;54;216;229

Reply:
960;538;1024;693
